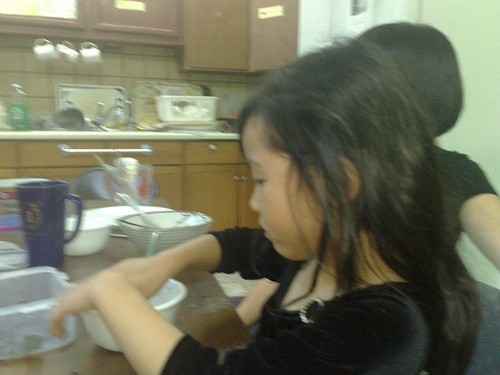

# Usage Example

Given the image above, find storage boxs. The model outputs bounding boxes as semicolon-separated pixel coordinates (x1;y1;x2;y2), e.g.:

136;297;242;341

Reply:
0;265;77;361
156;95;220;122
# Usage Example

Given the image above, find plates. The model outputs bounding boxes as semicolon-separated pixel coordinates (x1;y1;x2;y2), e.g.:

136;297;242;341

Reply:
115;210;215;254
82;206;176;220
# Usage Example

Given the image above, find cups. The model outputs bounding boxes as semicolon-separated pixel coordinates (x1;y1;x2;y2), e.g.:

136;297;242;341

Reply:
15;180;83;268
31;37;103;64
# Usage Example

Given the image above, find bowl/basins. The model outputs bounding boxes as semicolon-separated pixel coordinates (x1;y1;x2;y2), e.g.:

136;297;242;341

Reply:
63;217;111;256
79;278;189;352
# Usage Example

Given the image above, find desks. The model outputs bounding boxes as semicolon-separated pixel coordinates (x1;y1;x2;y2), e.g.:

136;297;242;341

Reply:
0;196;262;375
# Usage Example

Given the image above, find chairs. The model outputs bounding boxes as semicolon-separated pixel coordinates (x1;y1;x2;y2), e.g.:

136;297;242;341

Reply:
224;276;500;375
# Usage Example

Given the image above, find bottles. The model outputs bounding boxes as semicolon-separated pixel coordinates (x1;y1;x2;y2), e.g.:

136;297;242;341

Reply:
8;81;24;130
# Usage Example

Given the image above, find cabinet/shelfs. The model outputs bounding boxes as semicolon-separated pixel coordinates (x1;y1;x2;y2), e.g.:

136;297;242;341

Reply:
0;0;300;72
0;131;262;231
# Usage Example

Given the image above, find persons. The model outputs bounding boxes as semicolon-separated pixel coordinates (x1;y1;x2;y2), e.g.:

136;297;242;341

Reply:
45;20;500;375
